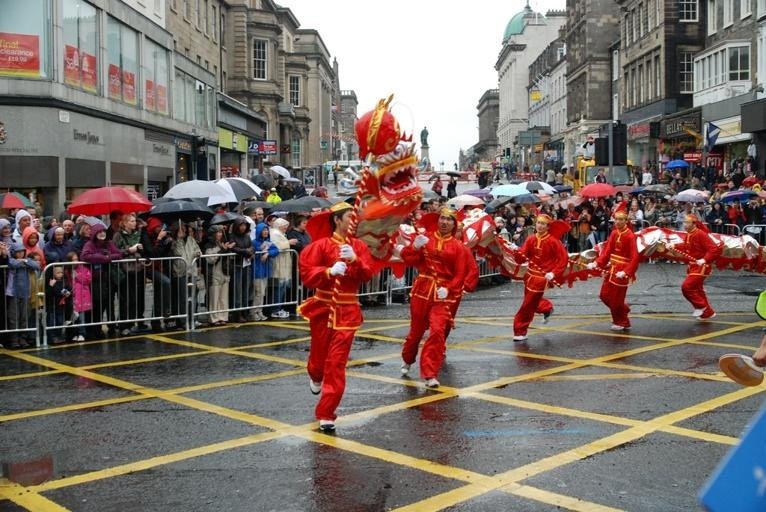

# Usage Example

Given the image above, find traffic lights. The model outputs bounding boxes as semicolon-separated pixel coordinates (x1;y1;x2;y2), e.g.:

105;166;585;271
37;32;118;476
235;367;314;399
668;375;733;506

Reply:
503;150;505;156
506;148;510;159
193;134;207;150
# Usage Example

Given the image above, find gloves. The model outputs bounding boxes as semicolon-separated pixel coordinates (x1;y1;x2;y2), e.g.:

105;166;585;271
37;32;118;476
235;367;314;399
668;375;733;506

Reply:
436;287;449;299
339;244;355;260
329;261;347;277
586;262;597;270
413;234;430;249
509;243;518;252
696;258;706;266
615;271;626;279
665;243;675;251
545;272;555;281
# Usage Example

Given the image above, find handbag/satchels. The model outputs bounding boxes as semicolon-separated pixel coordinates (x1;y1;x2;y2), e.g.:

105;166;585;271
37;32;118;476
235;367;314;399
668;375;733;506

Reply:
120;252;145;273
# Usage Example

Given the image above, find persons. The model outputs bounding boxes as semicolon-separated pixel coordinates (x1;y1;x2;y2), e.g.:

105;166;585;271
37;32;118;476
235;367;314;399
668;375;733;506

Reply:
581;136;596;161
420;126;429;145
747;139;757;159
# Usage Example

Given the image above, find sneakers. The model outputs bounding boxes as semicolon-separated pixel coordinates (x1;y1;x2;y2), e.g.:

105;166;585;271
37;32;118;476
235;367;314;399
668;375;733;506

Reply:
309;379;322;395
424;378;440;388
107;321;176;336
512;334;528;341
184;318;228;328
400;358;410;376
610;324;632;331
691;306;717;321
237;309;291;322
7;333;85;349
319;420;336;432
542;308;554;324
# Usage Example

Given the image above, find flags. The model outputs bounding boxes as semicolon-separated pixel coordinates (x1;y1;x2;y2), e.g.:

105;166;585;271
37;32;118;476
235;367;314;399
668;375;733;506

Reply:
703;122;720;152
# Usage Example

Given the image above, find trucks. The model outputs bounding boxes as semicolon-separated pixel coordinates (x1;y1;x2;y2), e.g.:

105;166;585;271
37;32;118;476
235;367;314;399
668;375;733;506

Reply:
554;154;638;200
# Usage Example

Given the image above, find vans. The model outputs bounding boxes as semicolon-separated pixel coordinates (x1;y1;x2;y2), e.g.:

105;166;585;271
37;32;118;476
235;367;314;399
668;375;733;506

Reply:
322;160;369;184
